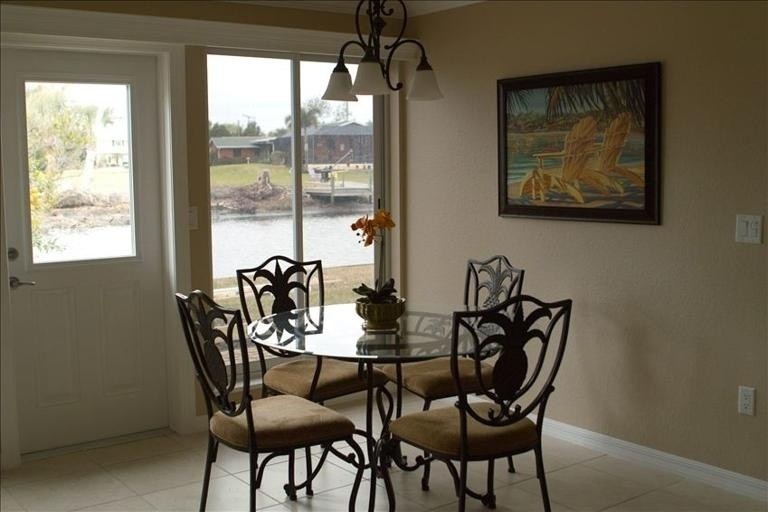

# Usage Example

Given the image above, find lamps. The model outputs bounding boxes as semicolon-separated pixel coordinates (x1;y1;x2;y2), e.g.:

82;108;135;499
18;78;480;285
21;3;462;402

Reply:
322;1;443;101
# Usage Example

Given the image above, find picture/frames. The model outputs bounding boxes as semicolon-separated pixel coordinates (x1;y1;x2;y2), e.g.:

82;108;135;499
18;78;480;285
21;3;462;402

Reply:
497;62;662;228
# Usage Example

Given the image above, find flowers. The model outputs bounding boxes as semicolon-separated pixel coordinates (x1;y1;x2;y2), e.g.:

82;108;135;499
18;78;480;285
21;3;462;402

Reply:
349;209;410;304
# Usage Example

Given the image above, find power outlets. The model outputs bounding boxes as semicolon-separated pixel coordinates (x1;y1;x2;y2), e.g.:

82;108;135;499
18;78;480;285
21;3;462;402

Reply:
737;386;755;415
